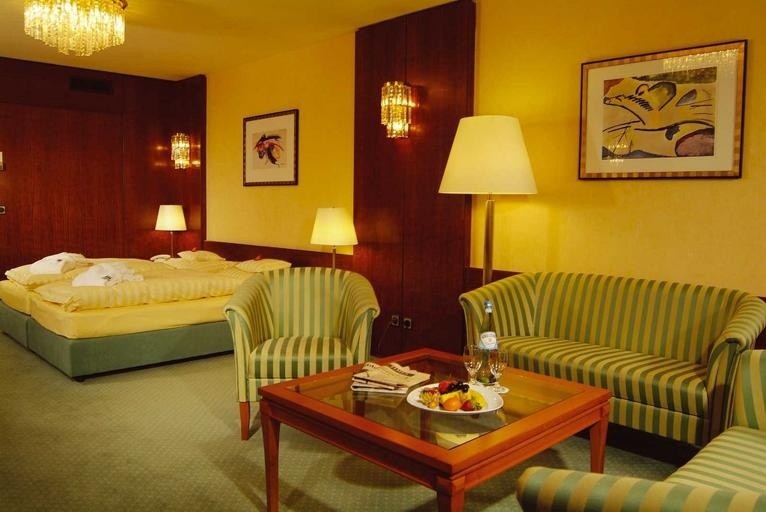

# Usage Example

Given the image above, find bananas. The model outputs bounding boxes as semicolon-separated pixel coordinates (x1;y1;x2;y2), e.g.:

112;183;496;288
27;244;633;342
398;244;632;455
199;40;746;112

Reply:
469;389;485;409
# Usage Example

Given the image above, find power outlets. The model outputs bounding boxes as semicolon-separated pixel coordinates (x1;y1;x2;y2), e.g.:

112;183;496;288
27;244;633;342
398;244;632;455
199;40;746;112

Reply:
391;315;399;326
403;318;411;329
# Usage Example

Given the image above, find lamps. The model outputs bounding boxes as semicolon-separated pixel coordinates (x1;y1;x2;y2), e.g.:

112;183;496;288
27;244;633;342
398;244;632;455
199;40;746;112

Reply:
380;81;420;139
309;208;358;268
437;115;538;286
170;132;190;170
24;1;125;58
154;205;186;258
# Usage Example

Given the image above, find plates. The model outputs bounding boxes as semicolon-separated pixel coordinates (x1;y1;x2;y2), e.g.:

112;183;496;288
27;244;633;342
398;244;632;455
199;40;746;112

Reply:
407;382;503;414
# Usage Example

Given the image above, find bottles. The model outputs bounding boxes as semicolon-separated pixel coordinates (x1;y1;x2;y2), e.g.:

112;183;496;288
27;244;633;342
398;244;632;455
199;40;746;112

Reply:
478;302;501;386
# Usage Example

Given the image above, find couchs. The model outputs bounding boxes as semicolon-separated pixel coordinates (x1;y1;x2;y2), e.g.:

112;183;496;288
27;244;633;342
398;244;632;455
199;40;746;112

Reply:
458;270;766;450
515;349;766;512
222;267;381;441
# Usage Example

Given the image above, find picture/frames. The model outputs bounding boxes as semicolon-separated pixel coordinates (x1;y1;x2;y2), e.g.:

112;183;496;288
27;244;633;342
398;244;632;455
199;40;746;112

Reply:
242;109;299;186
577;39;747;181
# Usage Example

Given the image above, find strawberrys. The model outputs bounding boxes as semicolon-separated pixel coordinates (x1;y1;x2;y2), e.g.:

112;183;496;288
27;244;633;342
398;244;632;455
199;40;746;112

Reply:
459;399;473;410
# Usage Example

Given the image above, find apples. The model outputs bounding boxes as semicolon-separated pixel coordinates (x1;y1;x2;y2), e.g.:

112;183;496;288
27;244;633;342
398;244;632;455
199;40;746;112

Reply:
437;379;455;393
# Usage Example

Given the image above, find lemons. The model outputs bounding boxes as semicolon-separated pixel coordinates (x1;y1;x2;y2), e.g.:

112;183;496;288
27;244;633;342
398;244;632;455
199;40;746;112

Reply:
443;396;460;411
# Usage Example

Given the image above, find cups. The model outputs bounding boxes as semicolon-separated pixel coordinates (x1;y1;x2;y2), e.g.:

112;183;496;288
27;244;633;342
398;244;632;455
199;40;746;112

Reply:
487;348;509;394
463;346;483;385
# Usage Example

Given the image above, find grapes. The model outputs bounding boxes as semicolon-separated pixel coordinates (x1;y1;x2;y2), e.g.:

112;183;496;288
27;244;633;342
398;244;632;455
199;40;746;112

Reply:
448;380;469;392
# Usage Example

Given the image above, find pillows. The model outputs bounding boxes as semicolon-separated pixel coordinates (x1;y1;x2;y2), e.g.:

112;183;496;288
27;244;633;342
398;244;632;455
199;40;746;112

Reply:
177;251;225;262
234;258;291;272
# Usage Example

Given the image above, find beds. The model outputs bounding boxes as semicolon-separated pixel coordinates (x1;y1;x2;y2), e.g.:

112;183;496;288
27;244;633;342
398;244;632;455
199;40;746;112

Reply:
0;241;353;382
